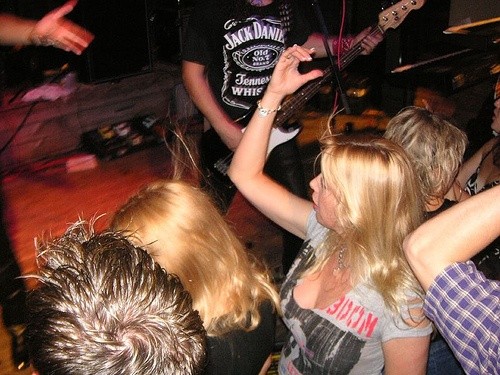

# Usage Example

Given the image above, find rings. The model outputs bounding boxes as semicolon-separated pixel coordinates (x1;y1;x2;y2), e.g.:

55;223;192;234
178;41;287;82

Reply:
282;50;287;54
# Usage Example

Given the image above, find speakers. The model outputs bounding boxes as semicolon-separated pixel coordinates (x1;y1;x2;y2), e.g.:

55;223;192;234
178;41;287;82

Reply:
54;0;153;85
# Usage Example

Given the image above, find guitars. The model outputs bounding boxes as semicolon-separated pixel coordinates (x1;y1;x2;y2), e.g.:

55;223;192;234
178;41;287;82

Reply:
197;0;426;215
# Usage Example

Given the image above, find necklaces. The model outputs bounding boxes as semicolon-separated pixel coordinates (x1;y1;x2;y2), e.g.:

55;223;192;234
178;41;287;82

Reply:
333;245;363;278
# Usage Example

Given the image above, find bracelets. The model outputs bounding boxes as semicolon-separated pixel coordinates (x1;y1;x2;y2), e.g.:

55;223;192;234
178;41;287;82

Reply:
256;100;281;116
458;183;463;201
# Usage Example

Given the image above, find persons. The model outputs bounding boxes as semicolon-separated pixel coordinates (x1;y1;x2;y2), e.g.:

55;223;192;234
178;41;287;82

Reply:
0;0;500;375
